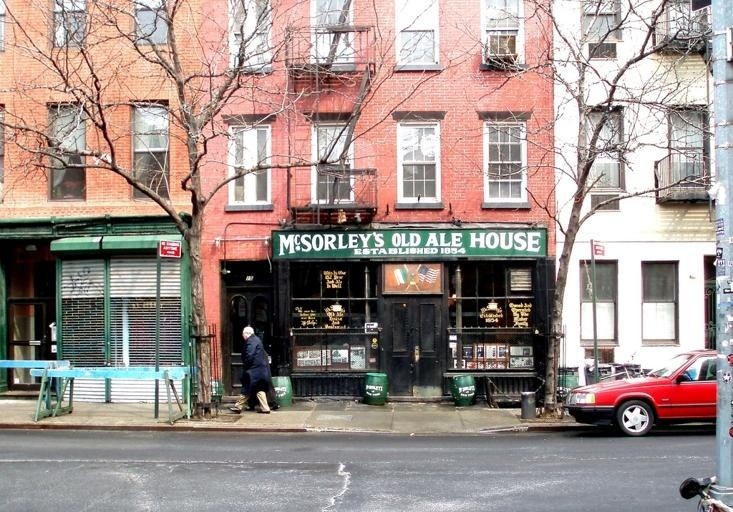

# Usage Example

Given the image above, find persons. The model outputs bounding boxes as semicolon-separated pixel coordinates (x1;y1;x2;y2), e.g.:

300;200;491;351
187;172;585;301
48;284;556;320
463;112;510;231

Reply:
230;326;272;413
703;499;733;512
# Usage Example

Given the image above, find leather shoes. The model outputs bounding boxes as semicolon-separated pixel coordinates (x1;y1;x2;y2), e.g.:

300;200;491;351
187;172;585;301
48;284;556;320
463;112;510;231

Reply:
227;407;241;414
256;410;270;414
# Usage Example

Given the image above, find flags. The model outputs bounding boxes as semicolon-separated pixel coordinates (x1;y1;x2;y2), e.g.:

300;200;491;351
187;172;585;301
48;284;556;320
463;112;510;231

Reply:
416;264;440;283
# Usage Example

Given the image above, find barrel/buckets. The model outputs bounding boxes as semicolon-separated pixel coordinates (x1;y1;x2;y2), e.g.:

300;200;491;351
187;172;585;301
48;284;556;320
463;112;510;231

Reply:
583;364;616;386
609;362;642;380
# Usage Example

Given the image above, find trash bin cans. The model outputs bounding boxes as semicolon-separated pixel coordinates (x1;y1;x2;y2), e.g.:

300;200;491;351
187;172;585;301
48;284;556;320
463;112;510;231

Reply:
584;367;612;386
615;363;641;380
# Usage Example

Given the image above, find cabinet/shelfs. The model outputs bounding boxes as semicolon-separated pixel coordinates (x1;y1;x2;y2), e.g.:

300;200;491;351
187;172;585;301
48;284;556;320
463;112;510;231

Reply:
289;327;379;373
447;326;536;372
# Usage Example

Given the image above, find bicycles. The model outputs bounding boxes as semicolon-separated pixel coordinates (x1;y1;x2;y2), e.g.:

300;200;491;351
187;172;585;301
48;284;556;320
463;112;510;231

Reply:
676;475;733;512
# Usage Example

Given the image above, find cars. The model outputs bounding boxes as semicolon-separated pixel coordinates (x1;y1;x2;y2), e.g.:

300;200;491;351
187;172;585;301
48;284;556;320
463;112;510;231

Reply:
568;348;717;435
